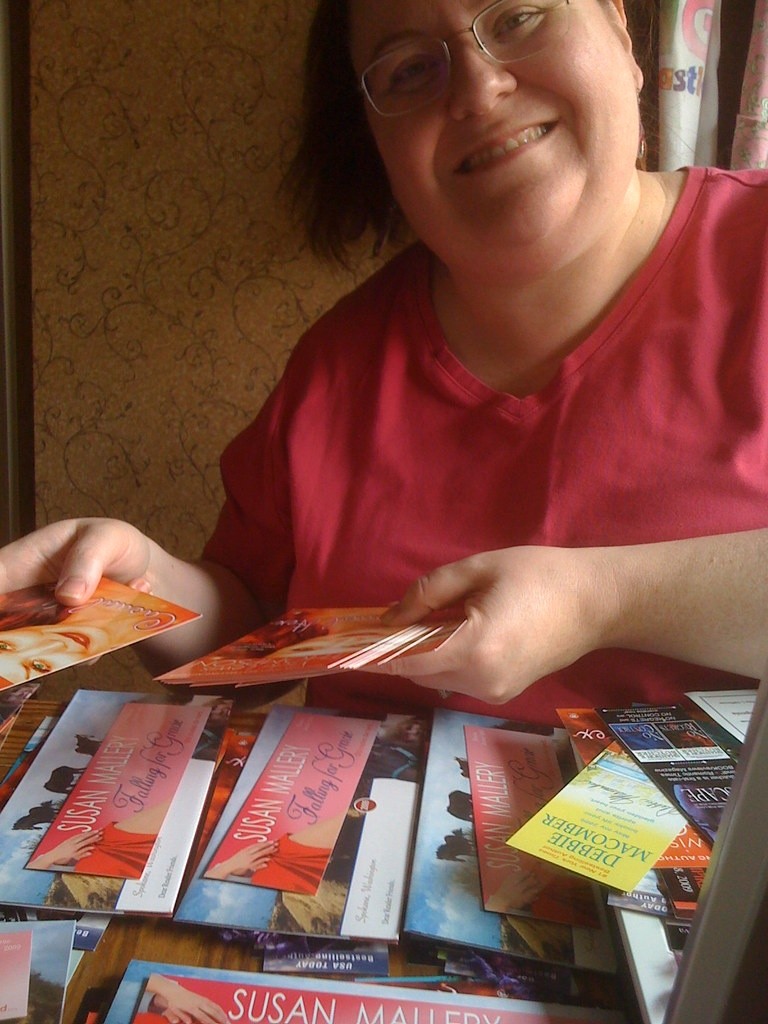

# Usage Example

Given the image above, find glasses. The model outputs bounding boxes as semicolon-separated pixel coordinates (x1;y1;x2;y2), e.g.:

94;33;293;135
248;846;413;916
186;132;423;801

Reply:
358;0;572;134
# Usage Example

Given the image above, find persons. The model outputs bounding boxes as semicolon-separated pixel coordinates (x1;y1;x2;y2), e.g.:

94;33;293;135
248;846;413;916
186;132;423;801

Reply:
205;813;343;893
25;798;174;880
265;624;395;658
1;0;768;728
485;866;600;927
0;578;166;689
131;975;231;1024
